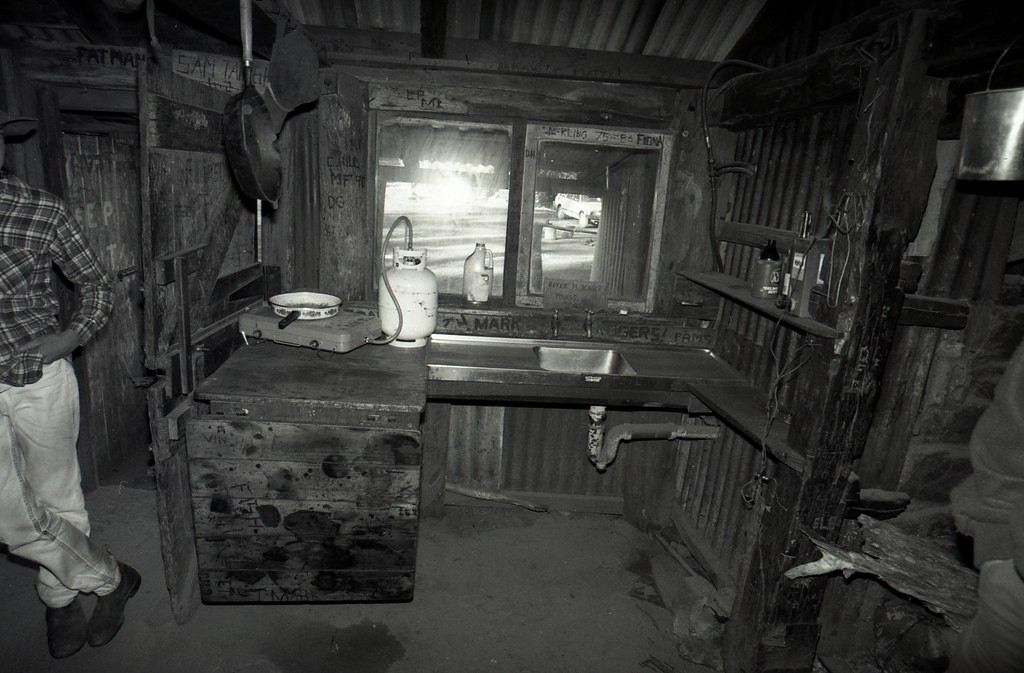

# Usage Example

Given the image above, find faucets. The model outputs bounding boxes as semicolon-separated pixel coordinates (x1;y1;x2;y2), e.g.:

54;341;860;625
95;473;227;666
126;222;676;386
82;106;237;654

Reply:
550;308;560;338
533;346;638;377
583;307;594;339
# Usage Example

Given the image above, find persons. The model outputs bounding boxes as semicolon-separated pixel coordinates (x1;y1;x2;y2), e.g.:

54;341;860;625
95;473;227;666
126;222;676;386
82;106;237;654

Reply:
0;109;141;658
947;339;1024;673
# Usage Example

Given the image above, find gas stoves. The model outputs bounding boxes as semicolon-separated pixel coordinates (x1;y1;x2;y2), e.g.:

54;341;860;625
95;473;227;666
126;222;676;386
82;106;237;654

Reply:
239;300;382;353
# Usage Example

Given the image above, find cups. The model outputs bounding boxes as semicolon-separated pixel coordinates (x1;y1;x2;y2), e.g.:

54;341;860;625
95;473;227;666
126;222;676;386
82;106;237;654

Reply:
751;260;782;298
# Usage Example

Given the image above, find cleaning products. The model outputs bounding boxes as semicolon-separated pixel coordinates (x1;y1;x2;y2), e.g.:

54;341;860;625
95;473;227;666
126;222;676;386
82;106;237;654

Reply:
460;243;494;309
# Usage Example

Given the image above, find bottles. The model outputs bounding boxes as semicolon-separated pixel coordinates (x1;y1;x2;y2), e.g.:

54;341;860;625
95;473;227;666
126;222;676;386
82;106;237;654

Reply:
461;242;494;302
749;240;780;262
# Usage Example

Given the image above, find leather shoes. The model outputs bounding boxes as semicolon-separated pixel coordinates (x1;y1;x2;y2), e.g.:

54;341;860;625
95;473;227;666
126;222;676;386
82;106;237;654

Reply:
46;596;87;659
87;561;141;647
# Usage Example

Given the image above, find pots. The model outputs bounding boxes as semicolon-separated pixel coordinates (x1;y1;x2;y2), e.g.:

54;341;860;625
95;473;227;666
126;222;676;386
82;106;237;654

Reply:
270;290;343;329
